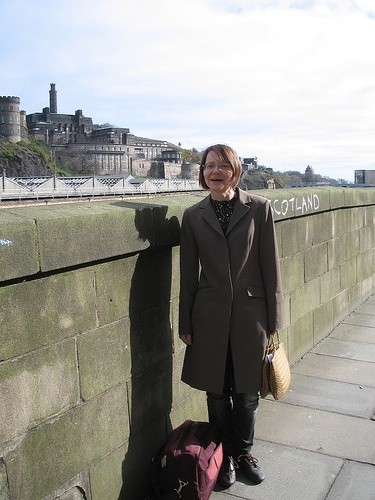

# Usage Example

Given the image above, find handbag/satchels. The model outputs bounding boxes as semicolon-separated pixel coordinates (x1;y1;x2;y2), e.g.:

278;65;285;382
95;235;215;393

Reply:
260;330;291;400
155;420;223;500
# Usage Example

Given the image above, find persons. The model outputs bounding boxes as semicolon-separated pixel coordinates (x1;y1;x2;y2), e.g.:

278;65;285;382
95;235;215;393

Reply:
179;144;284;487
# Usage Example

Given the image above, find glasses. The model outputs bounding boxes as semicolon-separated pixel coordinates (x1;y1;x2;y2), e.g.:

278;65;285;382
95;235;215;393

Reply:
202;163;231;170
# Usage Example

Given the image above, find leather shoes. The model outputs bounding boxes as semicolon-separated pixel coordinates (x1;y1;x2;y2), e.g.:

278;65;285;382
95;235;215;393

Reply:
235;454;266;482
220;456;239;484
